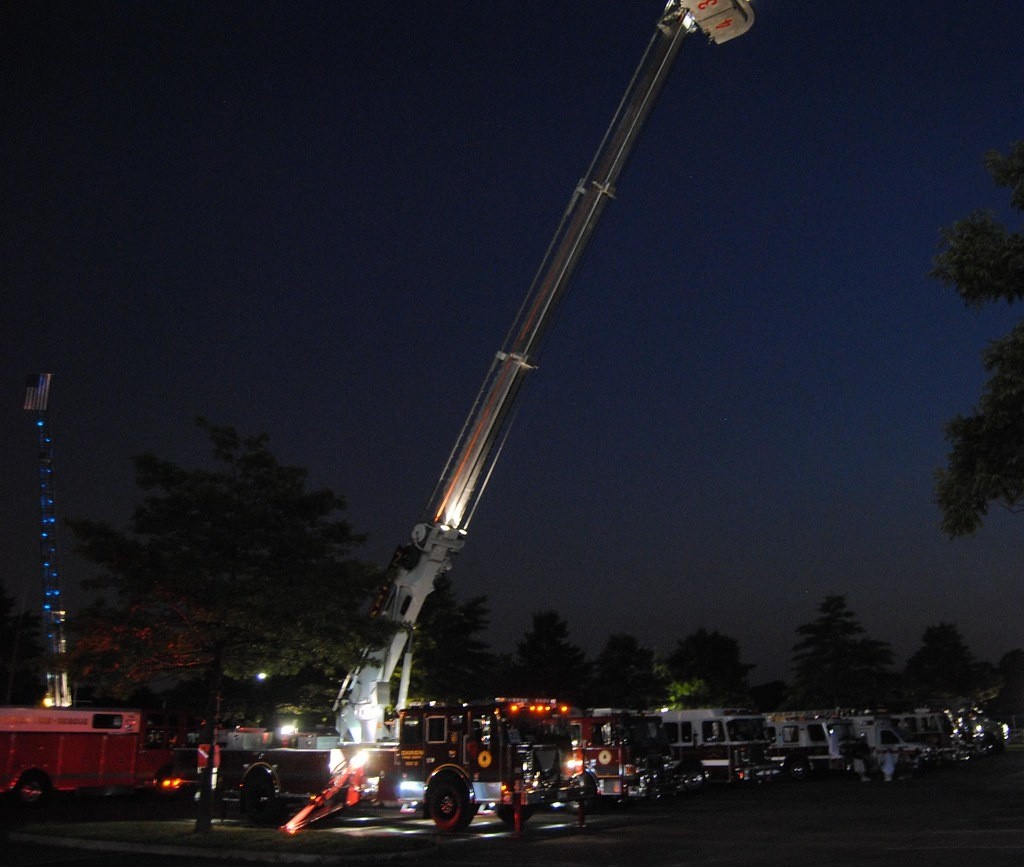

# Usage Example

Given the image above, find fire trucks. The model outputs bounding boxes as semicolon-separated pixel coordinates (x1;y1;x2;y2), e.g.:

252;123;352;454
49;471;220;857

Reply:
1;1;1023;835
